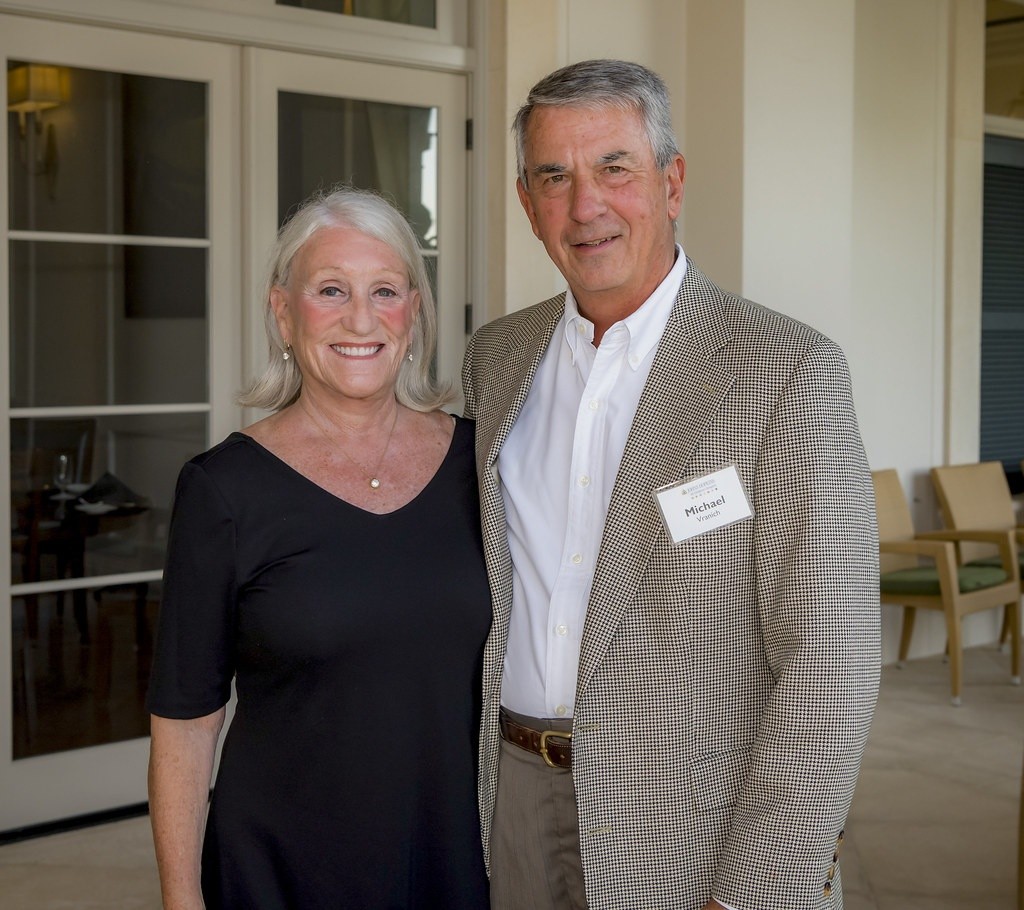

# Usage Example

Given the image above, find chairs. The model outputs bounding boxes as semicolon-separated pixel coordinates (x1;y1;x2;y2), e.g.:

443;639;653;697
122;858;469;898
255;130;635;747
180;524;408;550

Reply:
869;460;1024;706
932;460;1024;661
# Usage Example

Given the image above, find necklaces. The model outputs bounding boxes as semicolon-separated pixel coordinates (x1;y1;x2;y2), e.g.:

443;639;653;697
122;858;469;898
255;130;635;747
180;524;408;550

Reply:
298;397;399;488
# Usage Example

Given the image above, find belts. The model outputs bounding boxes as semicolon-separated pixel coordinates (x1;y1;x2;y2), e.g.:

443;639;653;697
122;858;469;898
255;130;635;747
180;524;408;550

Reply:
497;716;574;770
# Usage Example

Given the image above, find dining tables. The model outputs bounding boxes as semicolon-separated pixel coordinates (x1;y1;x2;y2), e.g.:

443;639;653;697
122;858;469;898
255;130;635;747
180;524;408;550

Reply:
9;480;152;702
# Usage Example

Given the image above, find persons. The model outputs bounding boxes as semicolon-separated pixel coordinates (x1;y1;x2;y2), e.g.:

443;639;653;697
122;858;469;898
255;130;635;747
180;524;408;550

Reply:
148;189;495;910
463;59;881;910
